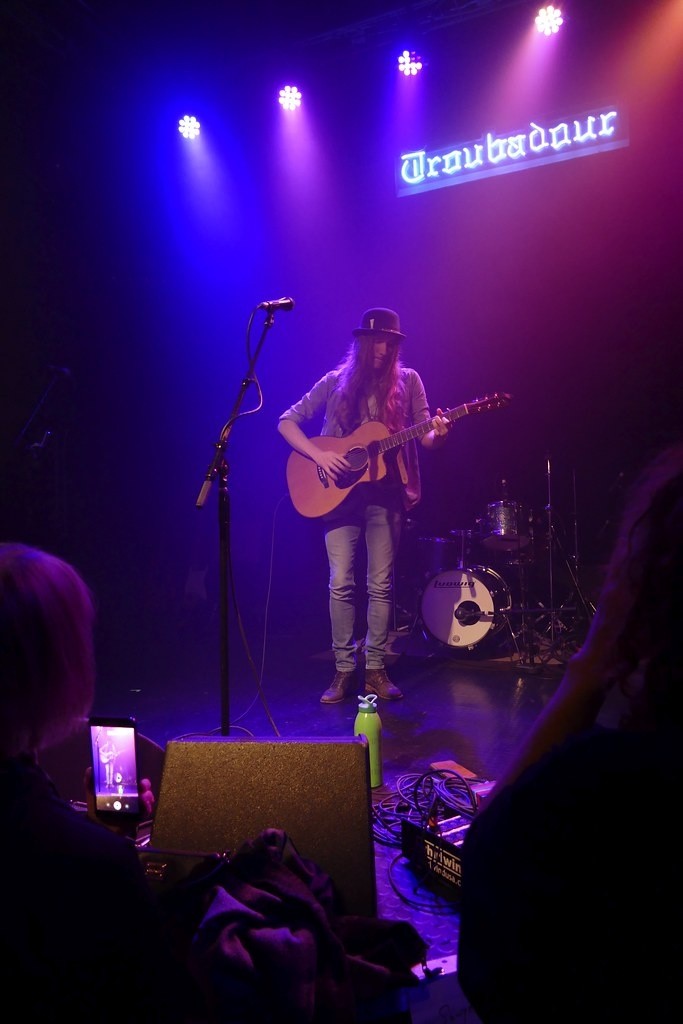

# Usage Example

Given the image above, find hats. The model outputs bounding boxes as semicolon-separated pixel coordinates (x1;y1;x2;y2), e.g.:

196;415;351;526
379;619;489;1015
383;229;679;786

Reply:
352;306;407;343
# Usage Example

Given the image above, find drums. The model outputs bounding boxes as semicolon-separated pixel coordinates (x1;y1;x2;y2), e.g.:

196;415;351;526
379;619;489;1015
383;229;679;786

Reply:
416;564;510;652
449;528;472;564
478;498;534;550
414;534;460;574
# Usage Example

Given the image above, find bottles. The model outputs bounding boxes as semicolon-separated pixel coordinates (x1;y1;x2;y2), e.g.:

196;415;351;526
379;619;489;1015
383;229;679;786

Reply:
354;694;382;787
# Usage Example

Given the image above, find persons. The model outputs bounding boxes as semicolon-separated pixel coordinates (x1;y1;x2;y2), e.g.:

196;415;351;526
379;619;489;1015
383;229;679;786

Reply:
456;450;683;1024
0;542;306;1024
100;733;124;788
278;308;456;703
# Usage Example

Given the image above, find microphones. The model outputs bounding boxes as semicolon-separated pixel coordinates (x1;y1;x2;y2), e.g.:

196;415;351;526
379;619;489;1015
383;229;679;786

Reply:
49;365;70;375
455;607;468;621
259;297;295;311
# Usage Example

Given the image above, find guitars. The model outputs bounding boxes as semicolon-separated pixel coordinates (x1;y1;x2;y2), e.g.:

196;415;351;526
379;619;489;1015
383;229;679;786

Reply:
285;389;515;522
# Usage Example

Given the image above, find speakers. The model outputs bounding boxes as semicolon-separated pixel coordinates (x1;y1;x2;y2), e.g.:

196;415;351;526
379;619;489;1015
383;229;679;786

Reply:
149;733;378;924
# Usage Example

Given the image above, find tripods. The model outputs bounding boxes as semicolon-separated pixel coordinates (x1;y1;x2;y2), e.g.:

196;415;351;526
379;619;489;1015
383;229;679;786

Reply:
497;459;598;656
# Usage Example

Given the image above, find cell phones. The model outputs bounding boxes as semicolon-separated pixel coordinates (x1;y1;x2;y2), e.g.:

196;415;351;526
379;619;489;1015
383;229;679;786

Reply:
88;716;143;831
430;760;477;780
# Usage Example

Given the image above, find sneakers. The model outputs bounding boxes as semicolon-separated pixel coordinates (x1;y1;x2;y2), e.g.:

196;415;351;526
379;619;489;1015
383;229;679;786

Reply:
365;662;402;699
319;669;355;704
